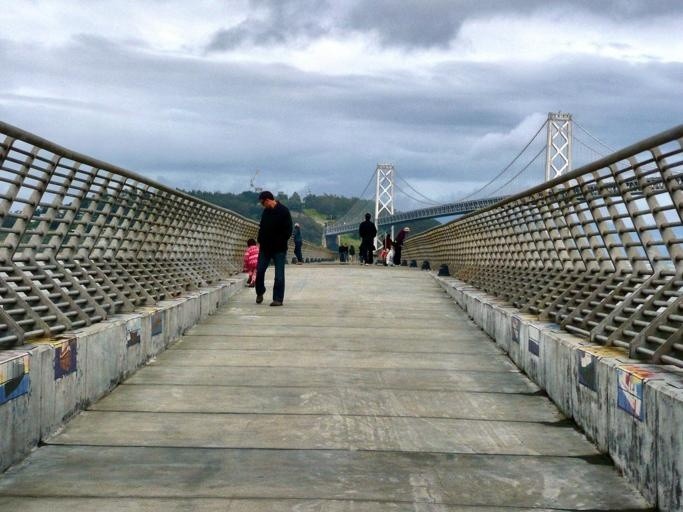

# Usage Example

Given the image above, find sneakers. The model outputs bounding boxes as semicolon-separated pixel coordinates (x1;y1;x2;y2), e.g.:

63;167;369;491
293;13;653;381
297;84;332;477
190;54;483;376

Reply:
255;288;283;306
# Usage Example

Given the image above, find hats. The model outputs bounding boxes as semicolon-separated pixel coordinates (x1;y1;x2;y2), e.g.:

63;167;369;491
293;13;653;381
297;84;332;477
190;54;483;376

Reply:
402;227;411;232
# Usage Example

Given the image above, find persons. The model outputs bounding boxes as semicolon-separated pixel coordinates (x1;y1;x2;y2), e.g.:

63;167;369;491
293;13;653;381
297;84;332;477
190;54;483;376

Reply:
381;234;396;251
393;226;410;265
335;243;397;268
255;191;292;305
242;238;260;287
358;213;376;266
293;222;303;266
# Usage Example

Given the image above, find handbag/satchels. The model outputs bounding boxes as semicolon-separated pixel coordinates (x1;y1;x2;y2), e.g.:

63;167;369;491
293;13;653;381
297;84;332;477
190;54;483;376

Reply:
385;246;396;266
